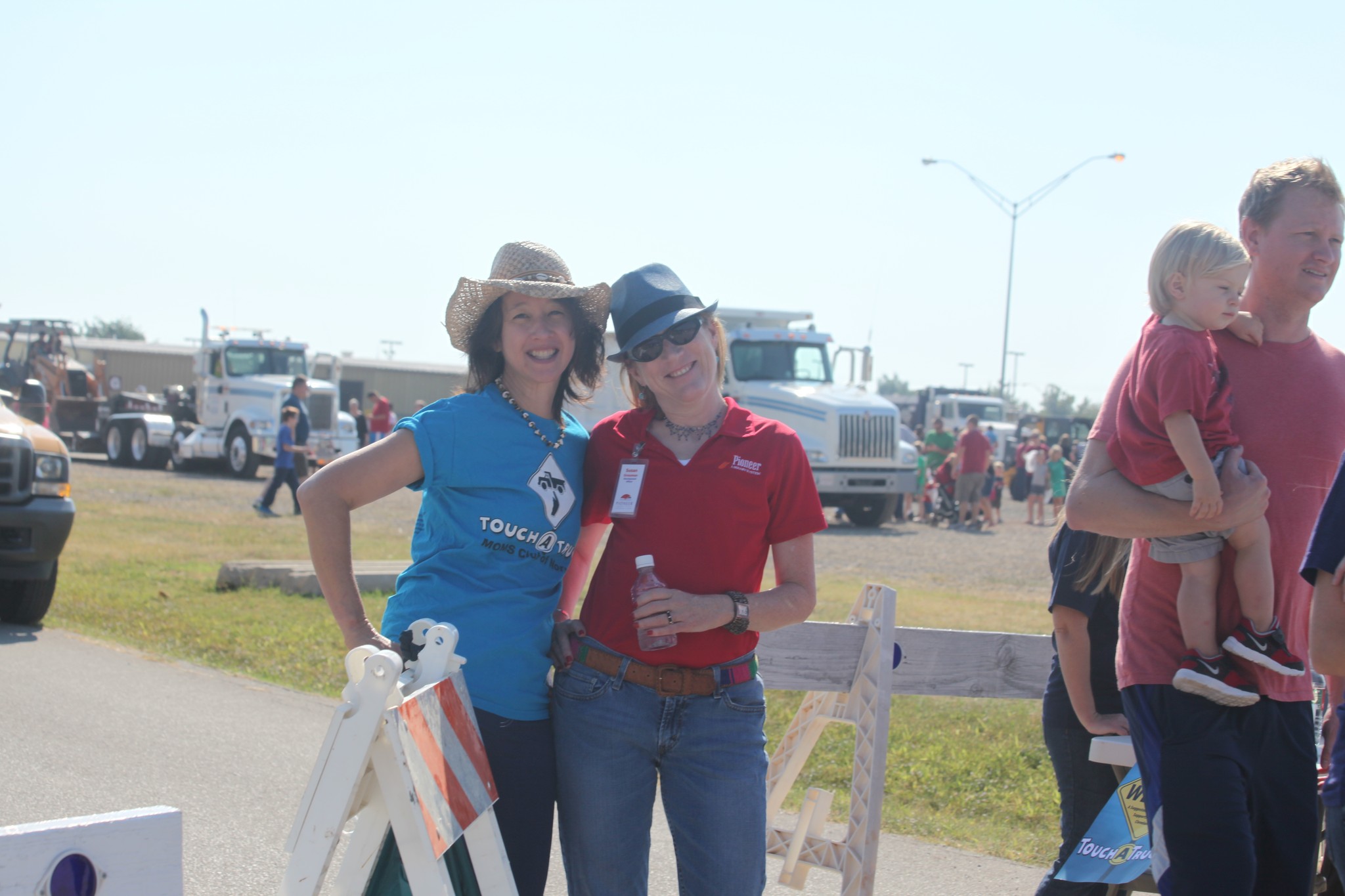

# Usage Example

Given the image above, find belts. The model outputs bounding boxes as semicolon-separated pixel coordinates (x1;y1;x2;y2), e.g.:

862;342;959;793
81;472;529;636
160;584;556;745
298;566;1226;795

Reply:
570;641;758;697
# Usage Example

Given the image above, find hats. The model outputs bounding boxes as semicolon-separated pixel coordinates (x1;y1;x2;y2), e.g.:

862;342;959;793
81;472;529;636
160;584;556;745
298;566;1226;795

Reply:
445;241;612;355
606;263;718;365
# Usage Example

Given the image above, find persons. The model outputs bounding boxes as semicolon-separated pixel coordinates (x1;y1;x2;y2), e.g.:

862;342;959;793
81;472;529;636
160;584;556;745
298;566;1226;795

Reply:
293;236;612;896
28;330;61;364
552;256;825;896
251;375;426;517
893;151;1345;896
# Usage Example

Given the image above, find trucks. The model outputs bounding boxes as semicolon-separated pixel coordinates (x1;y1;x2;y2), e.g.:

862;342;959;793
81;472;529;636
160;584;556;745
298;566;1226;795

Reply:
560;307;923;529
0;397;75;627
890;388;1021;462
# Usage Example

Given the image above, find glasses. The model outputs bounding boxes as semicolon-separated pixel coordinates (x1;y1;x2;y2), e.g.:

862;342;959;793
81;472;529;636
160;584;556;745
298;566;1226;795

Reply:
624;315;705;363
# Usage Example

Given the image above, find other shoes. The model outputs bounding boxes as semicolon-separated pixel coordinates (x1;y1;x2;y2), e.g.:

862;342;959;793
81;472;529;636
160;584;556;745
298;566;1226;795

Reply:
252;502;278;519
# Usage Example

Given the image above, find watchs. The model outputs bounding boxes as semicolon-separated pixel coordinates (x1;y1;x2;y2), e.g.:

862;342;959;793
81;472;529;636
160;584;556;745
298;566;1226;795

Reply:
726;588;751;635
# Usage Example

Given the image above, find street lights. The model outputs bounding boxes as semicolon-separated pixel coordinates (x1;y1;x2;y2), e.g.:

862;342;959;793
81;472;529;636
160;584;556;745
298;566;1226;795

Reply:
920;149;1125;400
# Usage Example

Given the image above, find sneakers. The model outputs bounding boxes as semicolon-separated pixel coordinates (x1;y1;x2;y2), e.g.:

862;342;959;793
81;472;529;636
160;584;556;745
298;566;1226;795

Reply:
1221;615;1305;677
1171;647;1260;707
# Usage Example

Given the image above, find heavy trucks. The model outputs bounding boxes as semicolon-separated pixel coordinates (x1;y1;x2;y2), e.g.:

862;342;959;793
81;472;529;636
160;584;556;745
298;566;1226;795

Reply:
100;309;363;481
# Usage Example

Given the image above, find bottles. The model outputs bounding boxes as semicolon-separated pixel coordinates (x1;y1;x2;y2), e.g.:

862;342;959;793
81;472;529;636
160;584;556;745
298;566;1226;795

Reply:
1306;658;1326;748
630;555;678;652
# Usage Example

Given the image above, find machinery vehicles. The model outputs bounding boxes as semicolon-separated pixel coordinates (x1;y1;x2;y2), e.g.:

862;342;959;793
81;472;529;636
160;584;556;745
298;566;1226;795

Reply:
0;315;105;437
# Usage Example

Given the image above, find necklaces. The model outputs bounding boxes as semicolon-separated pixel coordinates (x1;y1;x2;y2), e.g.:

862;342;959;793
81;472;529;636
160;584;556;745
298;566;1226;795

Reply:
495;375;567;449
662;405;723;442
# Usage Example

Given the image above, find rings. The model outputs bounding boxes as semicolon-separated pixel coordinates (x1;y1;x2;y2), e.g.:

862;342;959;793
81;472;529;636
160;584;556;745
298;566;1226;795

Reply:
667;611;674;624
